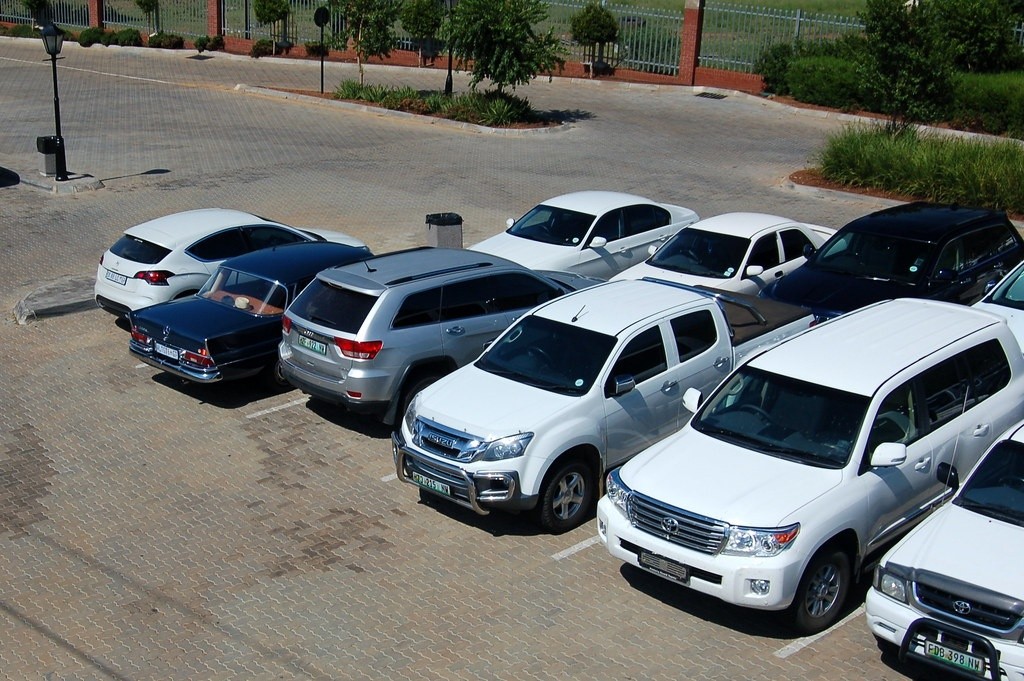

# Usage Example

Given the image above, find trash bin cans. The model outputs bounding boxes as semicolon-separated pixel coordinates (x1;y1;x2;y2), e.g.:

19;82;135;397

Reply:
37;135;57;177
425;212;462;247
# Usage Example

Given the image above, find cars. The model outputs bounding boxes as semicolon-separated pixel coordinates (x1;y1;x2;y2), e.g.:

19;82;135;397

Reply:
864;418;1024;681
972;261;1023;355
93;207;377;390
468;189;700;281
609;211;849;298
618;15;646;28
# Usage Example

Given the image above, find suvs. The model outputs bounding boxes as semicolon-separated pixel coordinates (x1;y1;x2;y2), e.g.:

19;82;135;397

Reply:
597;297;1024;636
275;244;609;428
757;201;1024;325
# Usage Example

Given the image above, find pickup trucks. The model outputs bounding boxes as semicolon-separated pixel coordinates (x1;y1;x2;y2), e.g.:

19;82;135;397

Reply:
389;277;818;533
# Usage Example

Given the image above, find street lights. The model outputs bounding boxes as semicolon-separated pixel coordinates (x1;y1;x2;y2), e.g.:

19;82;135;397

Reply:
39;19;70;181
